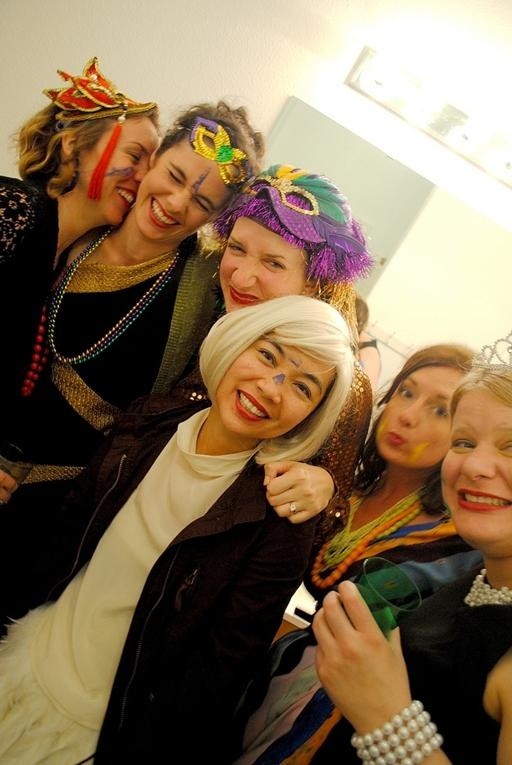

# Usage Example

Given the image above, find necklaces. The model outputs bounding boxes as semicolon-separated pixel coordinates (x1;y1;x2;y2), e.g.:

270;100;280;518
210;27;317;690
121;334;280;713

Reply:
462;565;511;610
308;482;431;590
48;221;186;366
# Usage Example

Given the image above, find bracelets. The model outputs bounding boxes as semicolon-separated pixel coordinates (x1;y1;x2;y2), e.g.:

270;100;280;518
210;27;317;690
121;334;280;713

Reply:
350;696;448;765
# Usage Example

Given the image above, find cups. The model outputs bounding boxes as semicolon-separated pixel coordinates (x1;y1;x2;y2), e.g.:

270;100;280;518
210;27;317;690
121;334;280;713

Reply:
344;557;423;634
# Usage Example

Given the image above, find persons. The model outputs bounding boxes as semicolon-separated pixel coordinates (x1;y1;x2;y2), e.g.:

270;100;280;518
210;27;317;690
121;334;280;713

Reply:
301;340;490;609
1;291;353;765
2;100;260;602
253;358;512;765
79;159;373;545
0;76;160;427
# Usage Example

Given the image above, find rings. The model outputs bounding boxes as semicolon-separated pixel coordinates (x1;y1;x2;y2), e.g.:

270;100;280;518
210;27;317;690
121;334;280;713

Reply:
289;503;298;513
